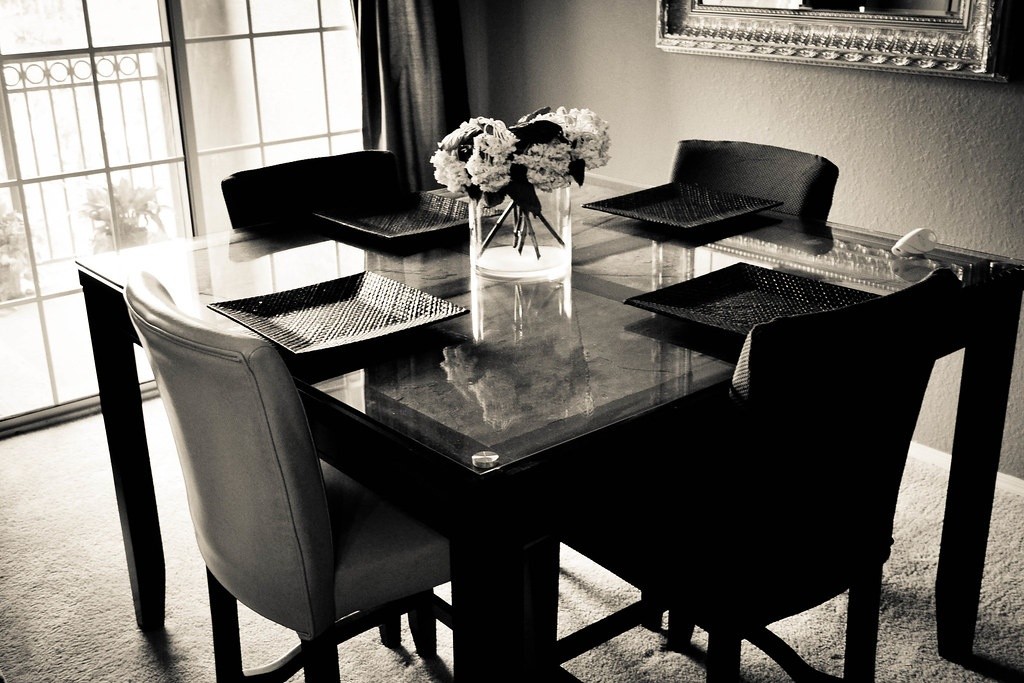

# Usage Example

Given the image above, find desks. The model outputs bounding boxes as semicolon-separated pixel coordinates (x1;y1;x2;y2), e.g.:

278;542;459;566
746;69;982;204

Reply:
76;176;1024;683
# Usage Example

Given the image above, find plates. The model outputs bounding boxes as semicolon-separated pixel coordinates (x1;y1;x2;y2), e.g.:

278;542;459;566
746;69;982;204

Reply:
621;262;885;338
206;270;472;355
313;191;508;238
581;181;786;229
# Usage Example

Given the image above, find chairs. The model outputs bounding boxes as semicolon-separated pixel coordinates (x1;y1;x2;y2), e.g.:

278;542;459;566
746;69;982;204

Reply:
123;139;962;683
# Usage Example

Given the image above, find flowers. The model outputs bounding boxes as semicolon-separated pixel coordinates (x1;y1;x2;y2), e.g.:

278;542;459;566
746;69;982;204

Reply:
429;106;611;259
440;330;591;432
79;175;166;241
1;204;39;273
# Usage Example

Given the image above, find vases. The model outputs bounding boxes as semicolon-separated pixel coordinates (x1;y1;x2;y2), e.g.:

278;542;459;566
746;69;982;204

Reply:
469;276;573;346
96;228;147;253
1;266;25;301
468;186;571;278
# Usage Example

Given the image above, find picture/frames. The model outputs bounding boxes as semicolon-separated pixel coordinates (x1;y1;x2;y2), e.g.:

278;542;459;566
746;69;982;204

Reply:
661;0;1012;84
